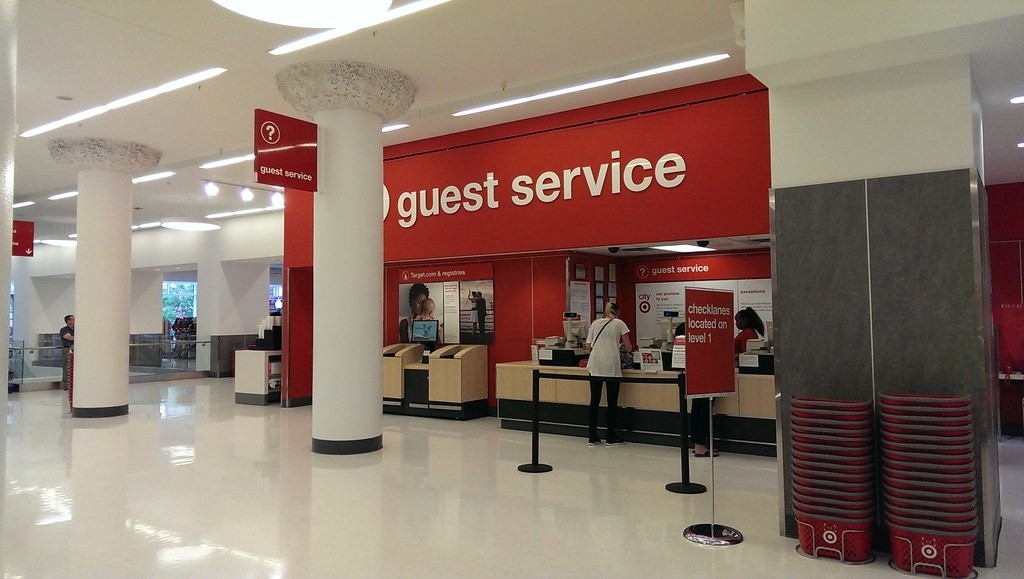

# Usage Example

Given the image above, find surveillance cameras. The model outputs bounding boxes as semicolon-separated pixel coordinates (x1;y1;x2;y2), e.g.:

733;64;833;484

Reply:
608;247;619;253
697;241;709;247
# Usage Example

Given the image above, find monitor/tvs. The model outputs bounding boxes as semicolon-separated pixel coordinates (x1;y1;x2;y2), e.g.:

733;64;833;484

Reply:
562;320;589;339
766;320;774;347
412;320;439;343
660;322;681;342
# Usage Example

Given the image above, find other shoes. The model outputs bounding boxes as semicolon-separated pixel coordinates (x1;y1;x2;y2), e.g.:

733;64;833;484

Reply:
605;438;626;446
588;439;601;446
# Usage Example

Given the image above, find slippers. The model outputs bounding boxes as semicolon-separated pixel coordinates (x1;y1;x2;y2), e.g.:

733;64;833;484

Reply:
695;450;719;458
692;447;718;454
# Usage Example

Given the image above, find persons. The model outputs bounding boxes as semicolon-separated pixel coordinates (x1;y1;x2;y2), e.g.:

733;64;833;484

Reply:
58;314;75;390
468;289;479;336
734;306;765;368
690;398;719;459
470;291;488;336
585;302;632;446
414;298;442;342
171;317;181;354
399;283;441;350
